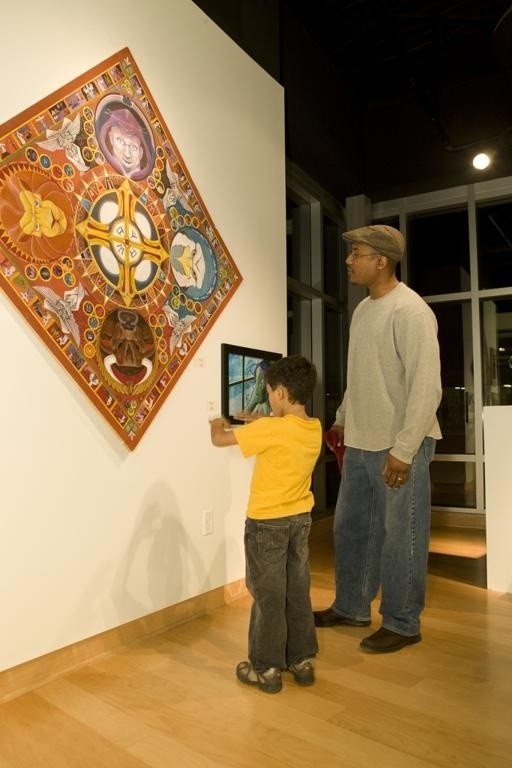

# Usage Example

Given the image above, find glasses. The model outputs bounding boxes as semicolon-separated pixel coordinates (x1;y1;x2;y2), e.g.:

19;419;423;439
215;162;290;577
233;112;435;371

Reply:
349;251;381;261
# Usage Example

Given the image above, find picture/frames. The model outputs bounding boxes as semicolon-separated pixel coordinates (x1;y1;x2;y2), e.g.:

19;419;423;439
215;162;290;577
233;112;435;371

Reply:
220;343;283;426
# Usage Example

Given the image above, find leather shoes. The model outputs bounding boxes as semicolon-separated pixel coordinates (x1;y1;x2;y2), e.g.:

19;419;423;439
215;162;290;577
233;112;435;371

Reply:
312;608;371;627
359;627;422;653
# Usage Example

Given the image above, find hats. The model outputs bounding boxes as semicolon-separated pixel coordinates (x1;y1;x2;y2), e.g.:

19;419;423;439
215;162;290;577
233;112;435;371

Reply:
342;225;405;262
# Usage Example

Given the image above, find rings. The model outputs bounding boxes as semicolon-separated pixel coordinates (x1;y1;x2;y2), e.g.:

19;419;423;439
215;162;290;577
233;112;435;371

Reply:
397;478;403;481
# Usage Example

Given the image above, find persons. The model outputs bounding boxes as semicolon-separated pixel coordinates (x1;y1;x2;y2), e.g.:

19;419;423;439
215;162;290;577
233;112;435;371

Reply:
313;224;444;654
109;127;144;172
209;355;323;694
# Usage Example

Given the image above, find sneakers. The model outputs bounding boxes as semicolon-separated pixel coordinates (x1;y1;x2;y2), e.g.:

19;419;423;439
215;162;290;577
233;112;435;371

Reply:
236;661;282;694
286;658;316;687
